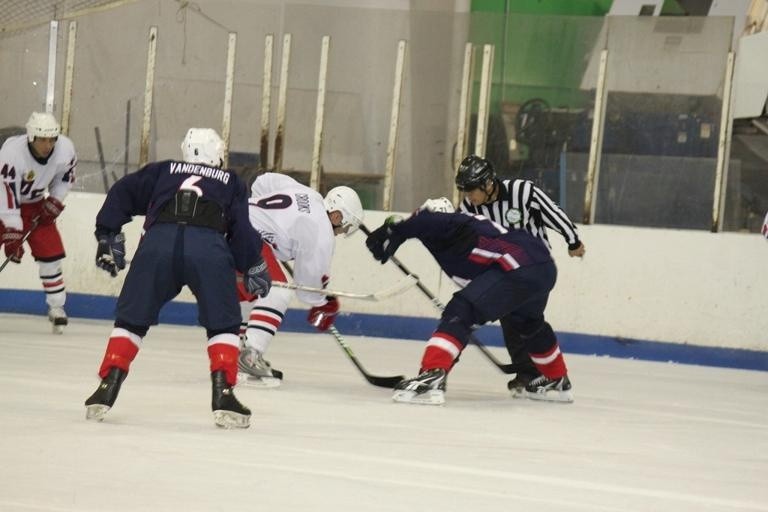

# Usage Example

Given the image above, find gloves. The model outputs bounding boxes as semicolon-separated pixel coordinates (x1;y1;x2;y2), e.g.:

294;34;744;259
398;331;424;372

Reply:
243;258;272;298
94;231;126;277
38;196;65;225
364;222;402;265
2;227;24;263
308;296;339;331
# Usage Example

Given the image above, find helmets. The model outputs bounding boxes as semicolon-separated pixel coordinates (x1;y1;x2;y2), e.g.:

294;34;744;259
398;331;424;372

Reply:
455;155;494;192
421;196;455;214
181;128;225;168
323;186;363;238
25;111;61;143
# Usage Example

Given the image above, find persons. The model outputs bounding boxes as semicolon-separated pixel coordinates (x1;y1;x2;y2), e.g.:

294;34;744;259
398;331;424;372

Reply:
366;197;572;393
455;155;584;390
0;111;79;325
85;127;272;415
235;173;364;378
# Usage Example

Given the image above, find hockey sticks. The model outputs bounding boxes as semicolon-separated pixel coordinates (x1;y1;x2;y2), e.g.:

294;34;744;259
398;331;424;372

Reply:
102;254;418;302
358;224;515;374
280;263;404;388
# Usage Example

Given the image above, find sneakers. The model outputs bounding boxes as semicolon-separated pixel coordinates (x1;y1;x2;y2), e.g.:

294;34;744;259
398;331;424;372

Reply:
396;367;447;392
507;371;571;392
48;306;67;325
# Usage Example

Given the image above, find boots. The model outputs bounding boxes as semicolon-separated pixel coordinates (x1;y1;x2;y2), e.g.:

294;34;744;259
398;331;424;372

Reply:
237;334;283;378
212;370;251;416
85;367;128;407
